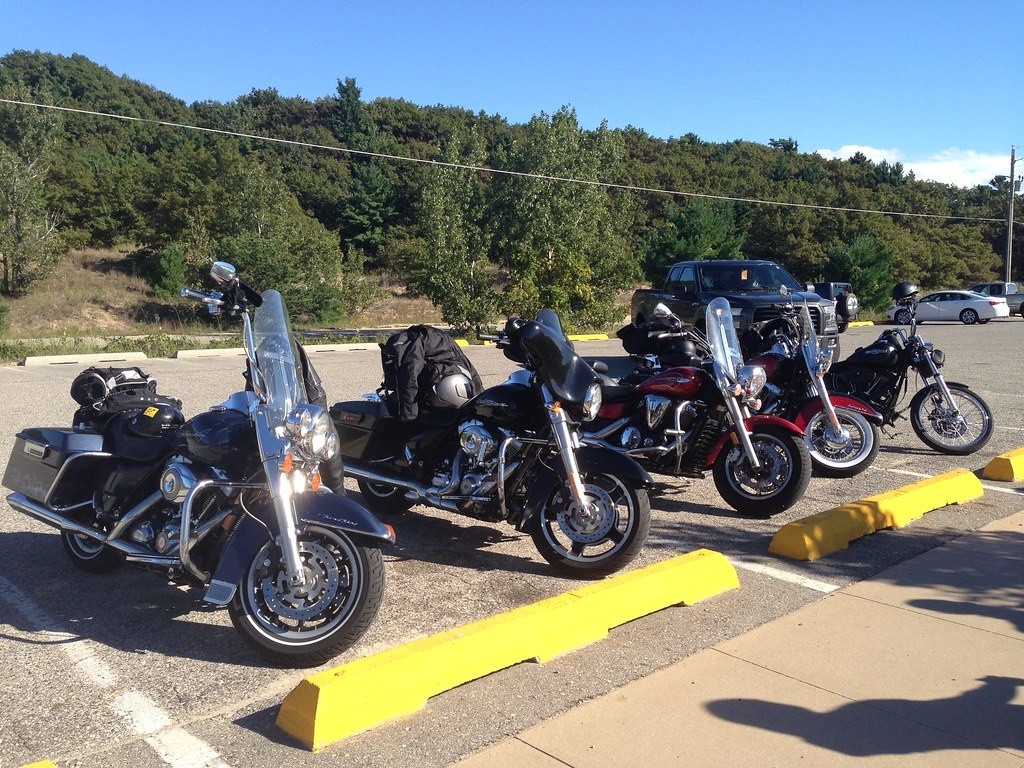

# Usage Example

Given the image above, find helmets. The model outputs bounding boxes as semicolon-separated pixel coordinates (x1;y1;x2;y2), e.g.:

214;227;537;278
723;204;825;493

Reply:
658;340;695;369
892;282;919;300
428;374;474;410
128;403;185;439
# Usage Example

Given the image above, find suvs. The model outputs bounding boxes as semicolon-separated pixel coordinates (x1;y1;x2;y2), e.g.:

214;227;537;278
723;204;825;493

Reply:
799;280;858;334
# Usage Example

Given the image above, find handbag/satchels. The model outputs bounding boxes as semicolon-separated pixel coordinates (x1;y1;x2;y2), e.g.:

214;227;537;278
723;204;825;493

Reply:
378;343;397;399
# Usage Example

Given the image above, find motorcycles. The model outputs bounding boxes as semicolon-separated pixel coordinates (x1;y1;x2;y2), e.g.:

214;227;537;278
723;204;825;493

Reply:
740;294;996;457
0;260;397;669
614;285;885;479
564;296;813;519
327;307;656;580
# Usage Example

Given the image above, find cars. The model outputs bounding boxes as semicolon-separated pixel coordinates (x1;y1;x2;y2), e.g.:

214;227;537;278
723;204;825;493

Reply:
886;290;1010;325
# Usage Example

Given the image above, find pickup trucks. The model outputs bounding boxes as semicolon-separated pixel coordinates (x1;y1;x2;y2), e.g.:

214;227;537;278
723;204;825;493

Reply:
959;281;1024;320
629;259;841;365
